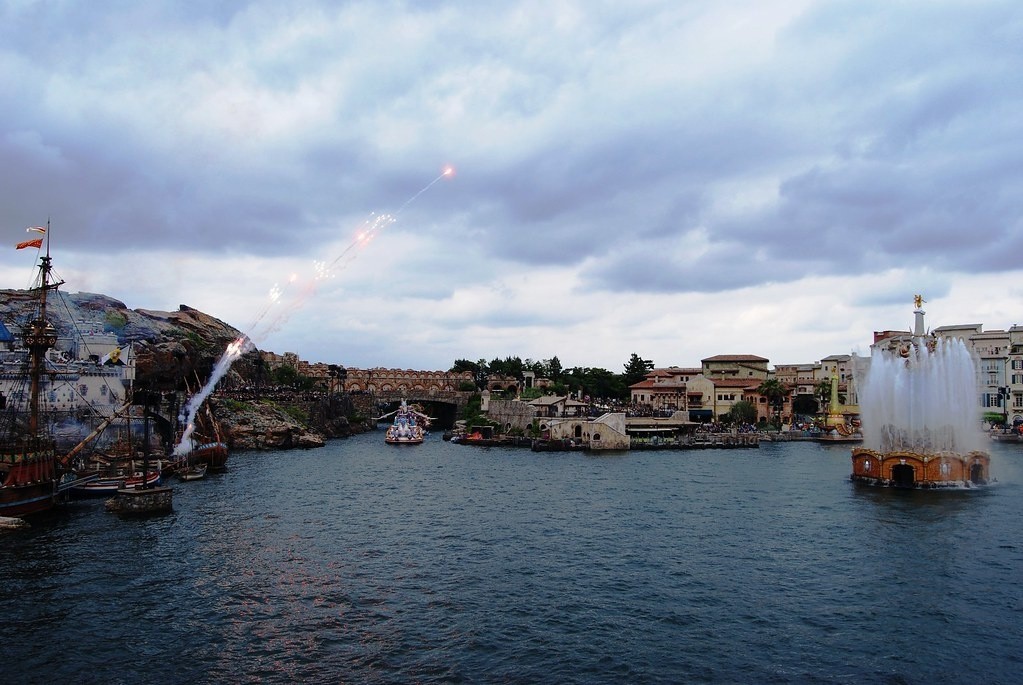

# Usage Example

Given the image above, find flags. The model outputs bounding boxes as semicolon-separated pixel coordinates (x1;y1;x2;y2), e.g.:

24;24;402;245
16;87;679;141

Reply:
26;224;46;235
16;238;43;249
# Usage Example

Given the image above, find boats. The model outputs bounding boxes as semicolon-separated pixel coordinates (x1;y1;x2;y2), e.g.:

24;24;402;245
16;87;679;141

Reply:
385;398;423;444
64;370;226;497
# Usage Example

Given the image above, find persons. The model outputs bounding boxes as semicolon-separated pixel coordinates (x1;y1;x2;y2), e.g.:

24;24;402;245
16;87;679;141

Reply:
771;413;819;431
4;354;87;365
548;390;656;417
207;382;323;401
691;419;761;436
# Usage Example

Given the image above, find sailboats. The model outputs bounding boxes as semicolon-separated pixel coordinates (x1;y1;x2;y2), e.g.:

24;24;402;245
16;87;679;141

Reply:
0;216;135;519
